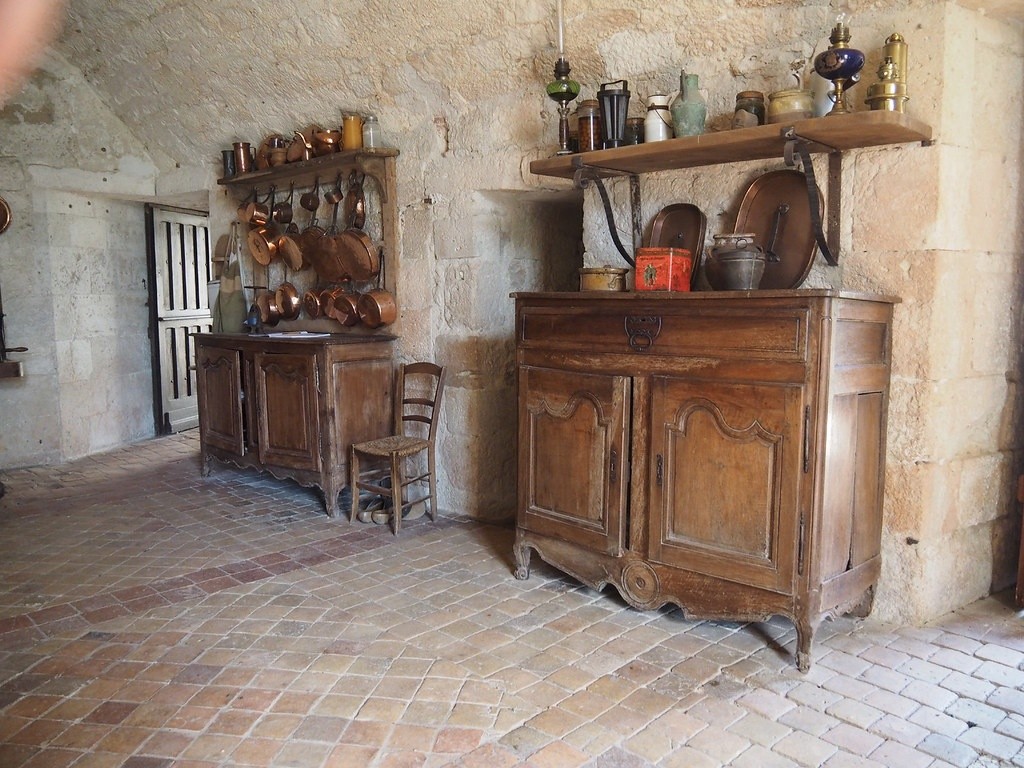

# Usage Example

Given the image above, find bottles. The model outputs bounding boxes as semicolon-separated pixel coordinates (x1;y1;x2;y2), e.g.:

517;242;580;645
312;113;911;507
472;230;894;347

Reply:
361;115;383;149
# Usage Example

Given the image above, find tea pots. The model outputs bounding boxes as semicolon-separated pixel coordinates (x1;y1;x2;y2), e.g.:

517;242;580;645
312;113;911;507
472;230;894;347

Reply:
294;128;343;157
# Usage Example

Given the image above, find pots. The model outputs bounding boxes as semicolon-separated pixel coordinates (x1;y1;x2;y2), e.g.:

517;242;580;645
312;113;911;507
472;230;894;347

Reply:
304;286;326;320
334;288;363;328
245;219;286;267
278;222;310;273
251;289;280;326
275;281;301;322
337;223;379;284
576;263;630;294
237;172;367;229
357;288;397;329
319;287;346;321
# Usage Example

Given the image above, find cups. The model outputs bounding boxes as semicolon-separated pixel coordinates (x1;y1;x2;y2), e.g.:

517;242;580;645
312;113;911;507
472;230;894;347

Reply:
341;112;363;150
222;142;257;179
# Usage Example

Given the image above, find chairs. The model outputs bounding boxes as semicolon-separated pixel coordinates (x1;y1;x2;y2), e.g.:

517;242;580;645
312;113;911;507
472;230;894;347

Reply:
347;362;449;537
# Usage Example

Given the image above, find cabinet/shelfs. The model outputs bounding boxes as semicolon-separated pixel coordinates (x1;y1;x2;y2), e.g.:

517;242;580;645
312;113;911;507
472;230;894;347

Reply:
508;289;904;674
187;330;401;519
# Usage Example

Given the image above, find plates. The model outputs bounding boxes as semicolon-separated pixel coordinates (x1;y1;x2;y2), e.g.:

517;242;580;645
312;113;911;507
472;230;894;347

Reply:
313;226;351;283
300;219;327;267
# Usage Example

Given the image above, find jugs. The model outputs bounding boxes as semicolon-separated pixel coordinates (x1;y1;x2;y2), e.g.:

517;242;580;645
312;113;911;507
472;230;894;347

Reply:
644;89;674;142
672;67;707;138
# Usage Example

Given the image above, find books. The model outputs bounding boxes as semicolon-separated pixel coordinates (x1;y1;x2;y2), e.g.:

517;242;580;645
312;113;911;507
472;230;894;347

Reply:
247;331;331;339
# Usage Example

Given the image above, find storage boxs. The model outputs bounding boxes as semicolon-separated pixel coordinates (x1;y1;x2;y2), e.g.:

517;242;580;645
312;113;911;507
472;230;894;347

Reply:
635;248;692;292
